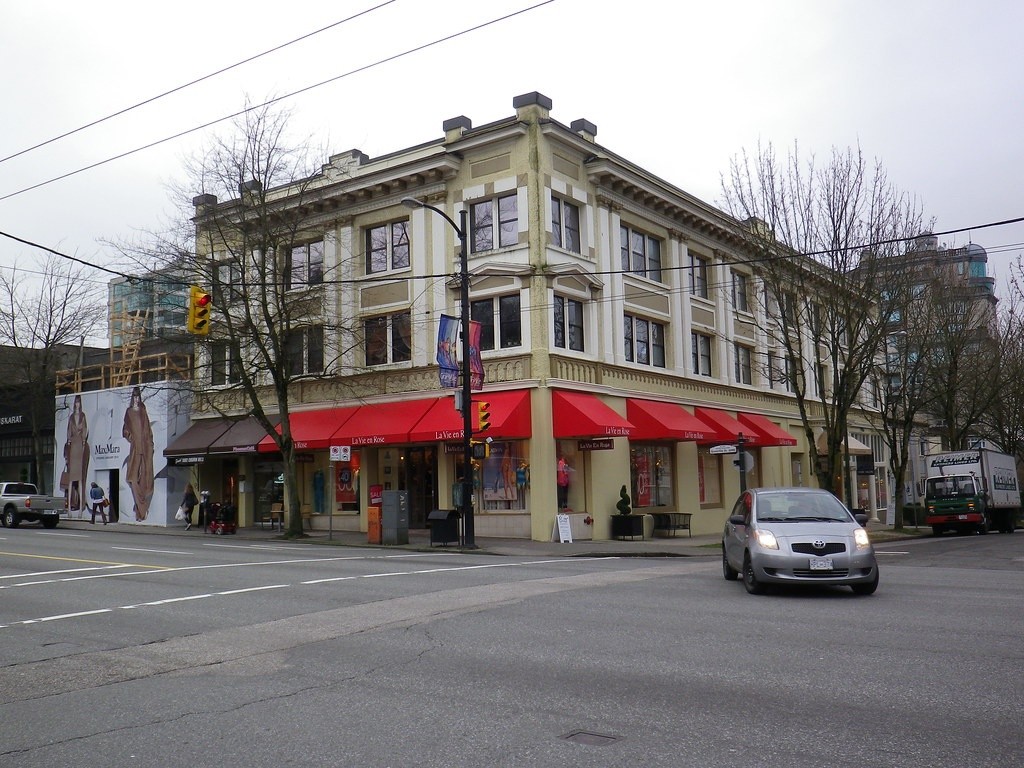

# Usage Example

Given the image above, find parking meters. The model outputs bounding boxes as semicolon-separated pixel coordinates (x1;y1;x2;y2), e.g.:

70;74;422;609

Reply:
201;491;211;533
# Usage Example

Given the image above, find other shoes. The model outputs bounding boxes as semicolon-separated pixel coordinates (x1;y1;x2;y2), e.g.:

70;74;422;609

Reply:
185;523;193;531
88;520;109;525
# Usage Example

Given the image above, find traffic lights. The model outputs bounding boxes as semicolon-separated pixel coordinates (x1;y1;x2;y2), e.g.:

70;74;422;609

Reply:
473;443;489;459
187;286;211;335
479;402;491;432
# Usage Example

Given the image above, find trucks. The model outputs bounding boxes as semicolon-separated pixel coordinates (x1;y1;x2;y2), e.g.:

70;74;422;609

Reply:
916;447;1022;538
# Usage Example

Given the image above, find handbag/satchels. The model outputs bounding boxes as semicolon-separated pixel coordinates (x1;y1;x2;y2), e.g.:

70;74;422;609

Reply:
102;495;110;508
174;505;186;521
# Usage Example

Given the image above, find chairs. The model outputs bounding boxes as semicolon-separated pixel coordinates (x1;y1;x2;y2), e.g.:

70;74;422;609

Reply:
261;503;283;531
651;512;693;538
300;504;313;532
611;513;644;542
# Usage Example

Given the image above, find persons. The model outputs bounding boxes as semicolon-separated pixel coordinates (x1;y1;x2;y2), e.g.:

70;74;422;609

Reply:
64;395;92;510
656;459;666;506
123;387;156;522
89;481;109;525
631;457;641;506
498;441;518;500
180;482;196;531
515;459;532;510
313;467;326;513
471;458;481;511
557;455;574;514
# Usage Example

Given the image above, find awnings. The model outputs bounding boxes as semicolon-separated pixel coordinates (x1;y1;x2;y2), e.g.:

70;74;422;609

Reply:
162;388;874;461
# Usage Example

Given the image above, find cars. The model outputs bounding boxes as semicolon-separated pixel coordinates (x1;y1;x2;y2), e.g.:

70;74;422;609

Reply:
722;486;880;596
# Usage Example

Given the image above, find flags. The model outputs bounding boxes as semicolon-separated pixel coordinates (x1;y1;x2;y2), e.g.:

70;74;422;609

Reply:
437;314;463;389
466;319;486;391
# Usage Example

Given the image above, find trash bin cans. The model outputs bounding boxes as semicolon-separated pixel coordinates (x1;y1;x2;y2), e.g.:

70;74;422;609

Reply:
428;510;461;547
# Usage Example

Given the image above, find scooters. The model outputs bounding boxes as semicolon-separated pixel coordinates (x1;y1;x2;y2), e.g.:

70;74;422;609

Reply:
210;502;237;535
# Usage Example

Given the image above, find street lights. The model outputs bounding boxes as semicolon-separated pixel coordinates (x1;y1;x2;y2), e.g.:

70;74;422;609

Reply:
839;331;910;515
401;196;478;551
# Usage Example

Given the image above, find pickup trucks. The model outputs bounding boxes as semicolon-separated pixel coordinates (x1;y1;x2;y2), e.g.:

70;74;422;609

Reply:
0;482;67;529
